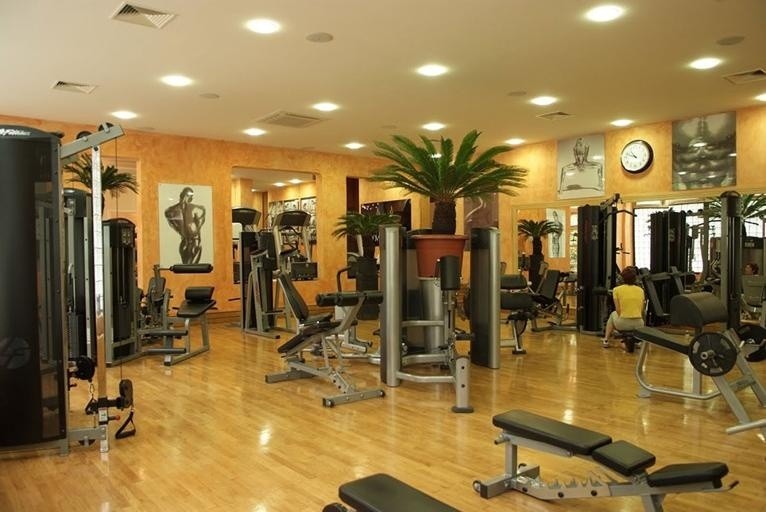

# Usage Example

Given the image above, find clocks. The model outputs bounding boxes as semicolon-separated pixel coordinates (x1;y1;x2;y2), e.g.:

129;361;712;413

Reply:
619;139;654;174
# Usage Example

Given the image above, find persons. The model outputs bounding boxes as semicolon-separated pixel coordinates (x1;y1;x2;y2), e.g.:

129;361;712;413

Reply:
162;185;207;266
744;262;759;275
601;267;646;349
560;137;604;191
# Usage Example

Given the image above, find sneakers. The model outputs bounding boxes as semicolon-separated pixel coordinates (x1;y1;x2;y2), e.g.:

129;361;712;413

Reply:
599;336;609;348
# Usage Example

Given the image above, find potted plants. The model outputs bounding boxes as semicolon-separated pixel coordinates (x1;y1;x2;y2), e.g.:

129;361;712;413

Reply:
698;191;765;237
67;153;136;224
518;219;560;281
354;129;562;320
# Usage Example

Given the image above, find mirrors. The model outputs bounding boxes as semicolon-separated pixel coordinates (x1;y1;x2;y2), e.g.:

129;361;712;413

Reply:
510;190;765;330
